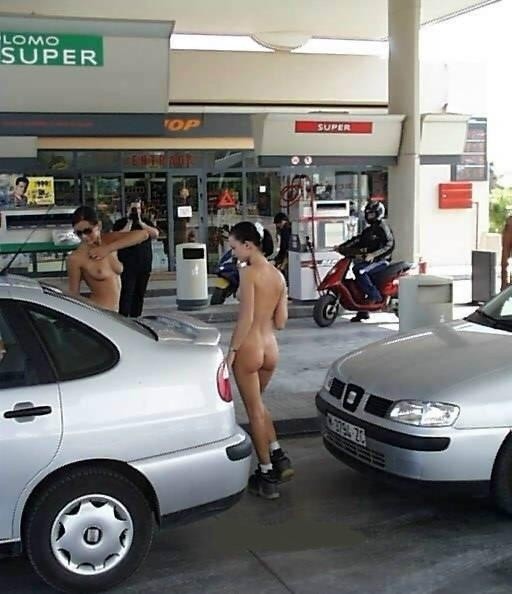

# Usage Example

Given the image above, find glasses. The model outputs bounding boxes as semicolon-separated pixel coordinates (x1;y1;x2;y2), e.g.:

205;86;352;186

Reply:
77;229;92;236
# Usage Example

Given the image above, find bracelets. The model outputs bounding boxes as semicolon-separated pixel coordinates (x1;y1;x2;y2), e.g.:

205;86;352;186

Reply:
229;346;238;352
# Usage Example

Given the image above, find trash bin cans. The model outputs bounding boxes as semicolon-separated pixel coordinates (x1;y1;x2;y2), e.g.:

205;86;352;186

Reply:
175;243;209;310
399;273;454;334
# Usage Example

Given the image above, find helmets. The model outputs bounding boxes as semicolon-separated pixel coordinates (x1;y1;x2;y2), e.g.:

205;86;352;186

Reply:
365;201;385;224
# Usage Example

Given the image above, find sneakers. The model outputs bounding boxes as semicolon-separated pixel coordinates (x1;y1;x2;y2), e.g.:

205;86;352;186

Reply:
248;455;295;499
350;312;370;322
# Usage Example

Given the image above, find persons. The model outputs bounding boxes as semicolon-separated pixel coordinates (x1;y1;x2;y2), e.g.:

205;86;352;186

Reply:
222;219;296;499
114;195;162;315
10;175;30;207
334;198;395;322
66;204;149;314
275;211;293;274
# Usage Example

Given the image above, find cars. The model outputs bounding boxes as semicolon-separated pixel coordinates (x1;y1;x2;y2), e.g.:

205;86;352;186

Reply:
0;271;254;593
315;281;511;517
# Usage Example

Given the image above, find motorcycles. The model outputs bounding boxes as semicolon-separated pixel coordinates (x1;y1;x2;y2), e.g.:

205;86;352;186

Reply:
312;246;416;328
211;223;310;305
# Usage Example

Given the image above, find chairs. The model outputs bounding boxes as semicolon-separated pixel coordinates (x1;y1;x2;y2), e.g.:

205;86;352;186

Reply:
25;318;61;383
